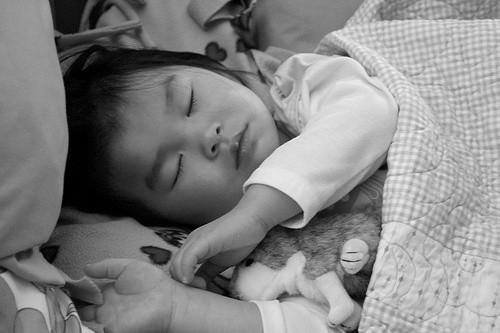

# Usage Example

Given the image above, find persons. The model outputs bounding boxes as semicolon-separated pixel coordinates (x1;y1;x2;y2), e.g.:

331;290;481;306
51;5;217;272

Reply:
52;18;500;333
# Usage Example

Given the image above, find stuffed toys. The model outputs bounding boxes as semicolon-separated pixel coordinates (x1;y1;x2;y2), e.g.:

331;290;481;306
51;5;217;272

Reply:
226;207;383;332
